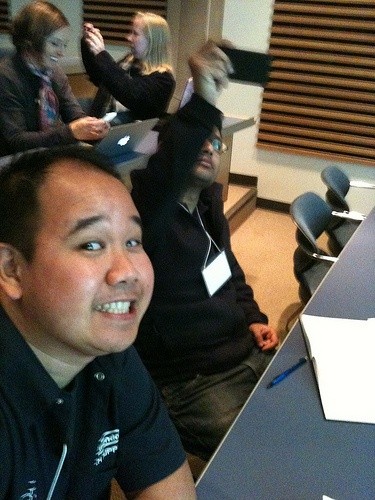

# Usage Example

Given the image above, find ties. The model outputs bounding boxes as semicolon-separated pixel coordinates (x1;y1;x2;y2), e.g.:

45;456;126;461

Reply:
26;60;60;129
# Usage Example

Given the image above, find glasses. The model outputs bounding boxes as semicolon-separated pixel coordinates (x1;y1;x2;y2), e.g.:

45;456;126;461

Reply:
208;137;228;153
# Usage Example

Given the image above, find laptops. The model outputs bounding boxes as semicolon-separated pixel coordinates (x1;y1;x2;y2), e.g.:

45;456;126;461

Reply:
91;118;160;159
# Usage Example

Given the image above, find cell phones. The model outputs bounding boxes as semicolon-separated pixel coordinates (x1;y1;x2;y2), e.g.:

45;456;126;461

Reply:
217;45;271;86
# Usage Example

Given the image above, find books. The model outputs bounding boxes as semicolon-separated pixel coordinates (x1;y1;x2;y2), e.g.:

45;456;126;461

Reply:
299;313;375;423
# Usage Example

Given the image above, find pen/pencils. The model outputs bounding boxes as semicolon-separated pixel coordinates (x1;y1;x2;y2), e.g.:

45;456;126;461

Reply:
266;355;308;388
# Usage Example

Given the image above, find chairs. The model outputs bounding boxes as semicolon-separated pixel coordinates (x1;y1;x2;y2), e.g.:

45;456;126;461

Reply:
320;165;375;255
290;192;361;304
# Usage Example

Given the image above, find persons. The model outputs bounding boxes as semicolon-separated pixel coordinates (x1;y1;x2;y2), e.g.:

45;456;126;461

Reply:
129;39;281;459
0;0;112;158
0;148;196;500
79;9;175;145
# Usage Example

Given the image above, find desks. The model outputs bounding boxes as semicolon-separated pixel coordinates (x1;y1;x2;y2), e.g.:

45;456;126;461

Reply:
211;113;257;202
193;204;374;499
